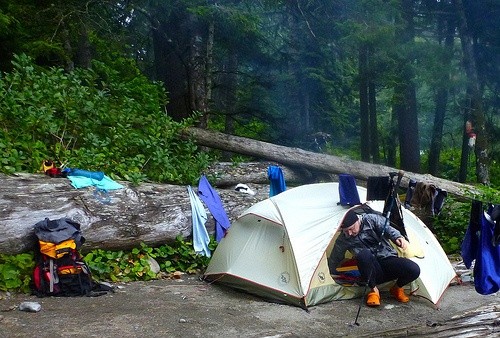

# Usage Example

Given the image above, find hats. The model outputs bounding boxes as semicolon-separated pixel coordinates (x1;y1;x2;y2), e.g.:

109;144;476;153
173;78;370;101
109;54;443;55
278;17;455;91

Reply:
340;209;359;228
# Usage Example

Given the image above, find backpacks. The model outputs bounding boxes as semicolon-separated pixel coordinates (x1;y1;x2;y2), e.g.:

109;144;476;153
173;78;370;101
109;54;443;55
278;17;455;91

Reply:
30;236;115;298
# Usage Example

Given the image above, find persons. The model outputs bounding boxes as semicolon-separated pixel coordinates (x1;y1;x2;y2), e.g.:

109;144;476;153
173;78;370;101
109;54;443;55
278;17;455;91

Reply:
327;209;420;306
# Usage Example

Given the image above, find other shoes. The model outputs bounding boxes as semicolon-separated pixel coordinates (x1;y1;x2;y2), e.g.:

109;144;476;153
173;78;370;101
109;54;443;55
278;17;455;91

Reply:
390;287;410;302
367;292;381;306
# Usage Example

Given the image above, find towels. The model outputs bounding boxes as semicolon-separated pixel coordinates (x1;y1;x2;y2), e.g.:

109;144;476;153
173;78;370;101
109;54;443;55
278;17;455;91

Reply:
198;176;232;243
339;174;360;206
267;166;286;198
389;229;425;258
187;185;211;258
404;179;447;217
367;176;390;201
67;175;124;190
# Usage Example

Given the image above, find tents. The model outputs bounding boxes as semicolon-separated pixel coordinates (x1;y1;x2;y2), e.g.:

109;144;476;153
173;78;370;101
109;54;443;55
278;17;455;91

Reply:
203;182;460;311
